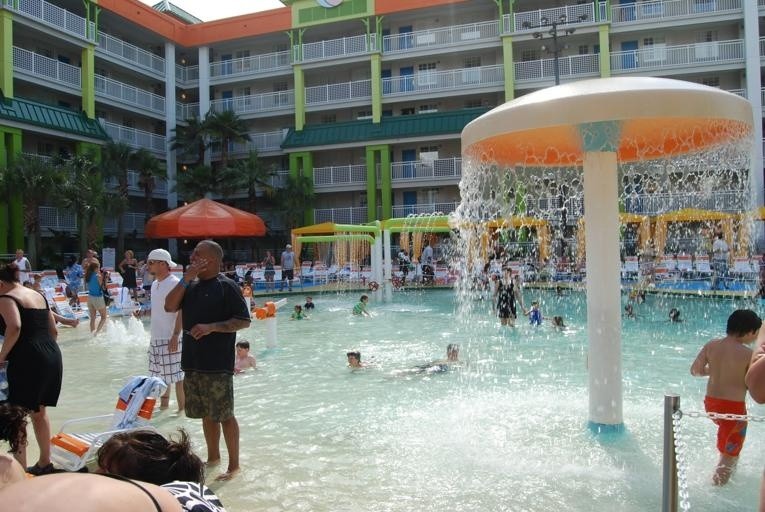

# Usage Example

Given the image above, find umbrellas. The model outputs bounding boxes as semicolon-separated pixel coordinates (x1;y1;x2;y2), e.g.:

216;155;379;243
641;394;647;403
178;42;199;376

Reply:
146;198;267;241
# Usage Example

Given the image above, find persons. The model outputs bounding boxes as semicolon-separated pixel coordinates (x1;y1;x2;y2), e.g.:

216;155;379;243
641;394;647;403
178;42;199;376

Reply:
688;308;762;486
383;342;468;383
226;244;314;319
619;228;764;324
345;348;380;369
352;295;375;317
398;240;585;330
2;241;255;511
744;318;765;404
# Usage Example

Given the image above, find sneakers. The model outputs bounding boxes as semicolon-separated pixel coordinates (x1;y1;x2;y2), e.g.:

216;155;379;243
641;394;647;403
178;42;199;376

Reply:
25;461;54;477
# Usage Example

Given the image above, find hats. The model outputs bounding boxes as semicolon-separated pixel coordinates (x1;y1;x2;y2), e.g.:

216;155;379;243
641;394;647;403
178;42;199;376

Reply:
147;249;177;269
286;244;293;249
33;273;44;280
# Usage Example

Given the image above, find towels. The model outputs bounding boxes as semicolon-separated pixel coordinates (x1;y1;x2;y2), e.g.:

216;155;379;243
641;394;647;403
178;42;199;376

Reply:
118;376;151;402
115;377;167;429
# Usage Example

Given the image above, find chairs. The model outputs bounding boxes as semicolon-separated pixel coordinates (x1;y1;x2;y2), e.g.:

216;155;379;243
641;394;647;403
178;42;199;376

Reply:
27;267;184;326
49;381;160;472
